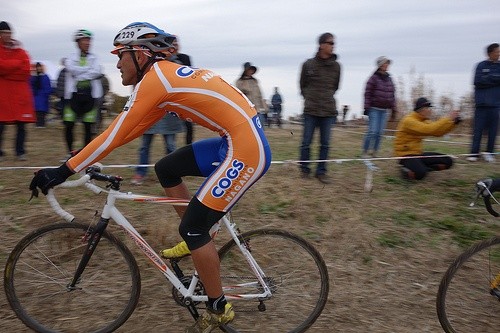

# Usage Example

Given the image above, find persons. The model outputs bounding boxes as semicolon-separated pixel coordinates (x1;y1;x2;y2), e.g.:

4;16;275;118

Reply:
270;86;283;128
0;20;37;160
57;30;109;164
30;62;51;127
363;56;396;155
390;96;463;181
297;31;341;185
466;43;500;163
131;34;194;184
29;21;272;333
235;61;264;127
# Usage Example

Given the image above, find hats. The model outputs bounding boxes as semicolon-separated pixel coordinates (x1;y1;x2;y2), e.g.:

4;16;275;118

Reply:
378;56;391;71
413;97;431;111
241;62;257;77
0;22;11;30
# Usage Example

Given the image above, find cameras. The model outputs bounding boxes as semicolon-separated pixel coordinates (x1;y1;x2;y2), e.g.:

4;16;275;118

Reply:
453;114;462;126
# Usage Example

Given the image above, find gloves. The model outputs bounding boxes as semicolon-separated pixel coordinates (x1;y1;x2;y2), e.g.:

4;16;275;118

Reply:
362;110;368;116
29;162;75;196
476;178;500;197
390;111;396;118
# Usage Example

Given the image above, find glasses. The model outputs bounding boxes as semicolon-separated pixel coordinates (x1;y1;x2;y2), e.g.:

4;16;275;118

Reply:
324;41;334;45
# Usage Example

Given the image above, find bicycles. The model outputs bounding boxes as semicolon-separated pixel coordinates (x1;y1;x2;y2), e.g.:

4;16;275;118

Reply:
3;149;329;333
436;178;500;333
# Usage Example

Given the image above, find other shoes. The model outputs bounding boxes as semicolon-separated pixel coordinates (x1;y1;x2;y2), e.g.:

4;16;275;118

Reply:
465;157;479;162
483;154;494;162
315;174;331;185
130;173;149;185
161;241;190;260
300;172;313;180
185;301;236;333
18;155;28;161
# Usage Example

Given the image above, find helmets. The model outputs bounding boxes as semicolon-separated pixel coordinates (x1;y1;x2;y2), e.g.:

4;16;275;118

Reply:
110;22;175;61
73;30;92;41
